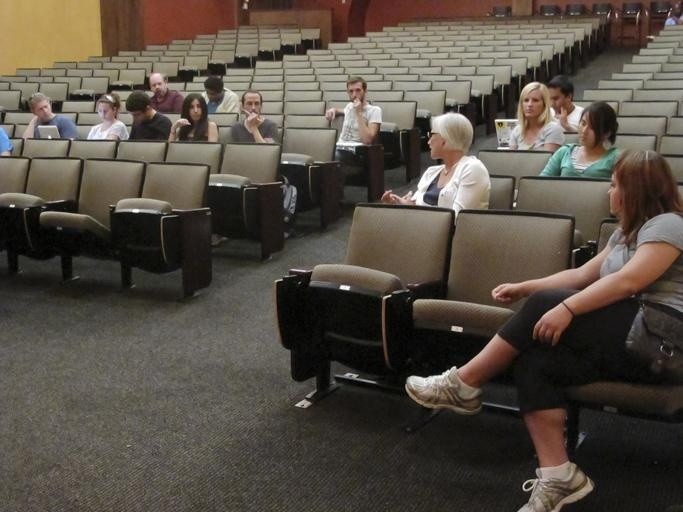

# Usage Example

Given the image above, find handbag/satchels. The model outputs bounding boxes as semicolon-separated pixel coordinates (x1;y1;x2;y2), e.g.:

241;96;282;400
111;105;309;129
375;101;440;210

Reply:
280;175;297;237
623;306;683;374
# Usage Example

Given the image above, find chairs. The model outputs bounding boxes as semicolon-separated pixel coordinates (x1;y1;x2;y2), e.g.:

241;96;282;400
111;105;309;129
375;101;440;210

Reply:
0;2;682;458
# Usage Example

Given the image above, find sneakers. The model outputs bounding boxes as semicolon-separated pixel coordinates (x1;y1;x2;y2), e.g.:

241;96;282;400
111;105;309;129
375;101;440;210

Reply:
406;365;482;415
516;463;594;512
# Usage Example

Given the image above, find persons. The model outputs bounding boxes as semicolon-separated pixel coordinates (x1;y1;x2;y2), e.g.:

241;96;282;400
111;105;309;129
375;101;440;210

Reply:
228;89;280;146
506;81;565;156
403;145;682;512
663;1;682;26
538;98;622;181
124;89;173;142
200;75;240;115
23;91;81;141
0;122;17;158
167;91;220;143
145;71;184;113
378;110;491;227
546;74;586;134
323;74;383;166
85;92;130;141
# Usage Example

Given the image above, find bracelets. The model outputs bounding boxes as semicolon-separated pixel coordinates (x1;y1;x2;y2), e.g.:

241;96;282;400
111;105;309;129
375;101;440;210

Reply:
170;127;176;135
561;301;577;319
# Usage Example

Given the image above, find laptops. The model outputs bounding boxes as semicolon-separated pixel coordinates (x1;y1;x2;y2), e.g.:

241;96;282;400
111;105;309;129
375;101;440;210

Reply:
494;119;518;147
38;125;61;139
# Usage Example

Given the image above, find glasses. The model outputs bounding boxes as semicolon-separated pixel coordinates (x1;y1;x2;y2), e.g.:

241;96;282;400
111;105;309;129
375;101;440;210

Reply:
427;132;440;137
